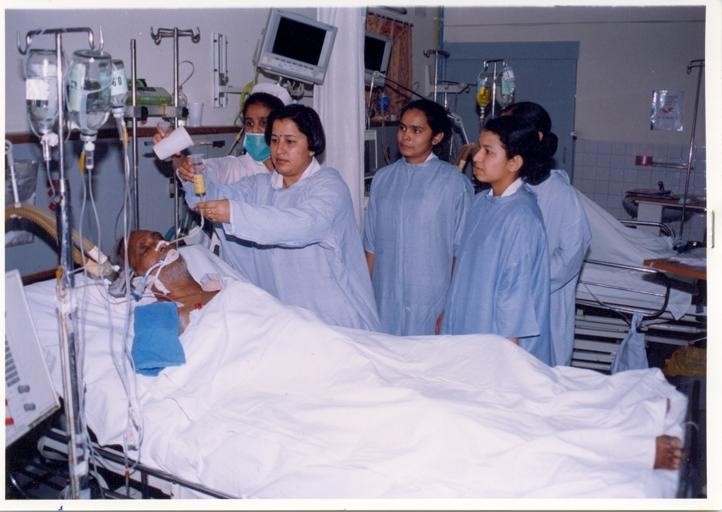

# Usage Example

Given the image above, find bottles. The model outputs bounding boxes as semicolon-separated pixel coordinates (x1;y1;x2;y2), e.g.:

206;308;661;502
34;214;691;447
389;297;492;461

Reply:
377;90;390;122
476;67;515;120
173;85;188;127
24;48;130;140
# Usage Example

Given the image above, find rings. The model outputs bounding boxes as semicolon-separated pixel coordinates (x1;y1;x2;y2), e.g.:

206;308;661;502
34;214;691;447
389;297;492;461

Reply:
208;209;212;213
209;215;213;219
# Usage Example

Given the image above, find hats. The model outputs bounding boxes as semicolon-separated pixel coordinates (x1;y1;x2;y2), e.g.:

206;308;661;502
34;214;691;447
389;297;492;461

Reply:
248;81;293;106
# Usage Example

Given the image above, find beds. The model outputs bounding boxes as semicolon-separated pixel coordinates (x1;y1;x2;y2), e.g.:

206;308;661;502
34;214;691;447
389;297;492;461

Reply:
4;260;706;497
563;219;706;371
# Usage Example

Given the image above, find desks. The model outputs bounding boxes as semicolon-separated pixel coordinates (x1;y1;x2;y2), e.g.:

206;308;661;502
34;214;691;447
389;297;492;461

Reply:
642;257;706;280
635;198;706;236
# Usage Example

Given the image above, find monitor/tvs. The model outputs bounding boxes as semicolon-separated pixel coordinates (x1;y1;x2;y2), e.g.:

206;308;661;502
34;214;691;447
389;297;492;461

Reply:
364;33;393;87
257;8;337;85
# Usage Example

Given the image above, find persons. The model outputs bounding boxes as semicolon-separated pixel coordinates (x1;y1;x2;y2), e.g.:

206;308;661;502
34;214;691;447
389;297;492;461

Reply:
438;115;557;367
178;102;382;332
360;98;475;337
502;101;591;365
114;229;681;470
152;82;294;261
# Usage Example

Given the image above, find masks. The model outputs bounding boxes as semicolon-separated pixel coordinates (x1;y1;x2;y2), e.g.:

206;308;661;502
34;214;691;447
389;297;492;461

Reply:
242;132;271;163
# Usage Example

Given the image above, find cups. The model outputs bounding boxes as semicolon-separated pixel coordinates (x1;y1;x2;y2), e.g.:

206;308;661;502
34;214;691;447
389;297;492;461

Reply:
152;126;194;161
189;101;204;128
